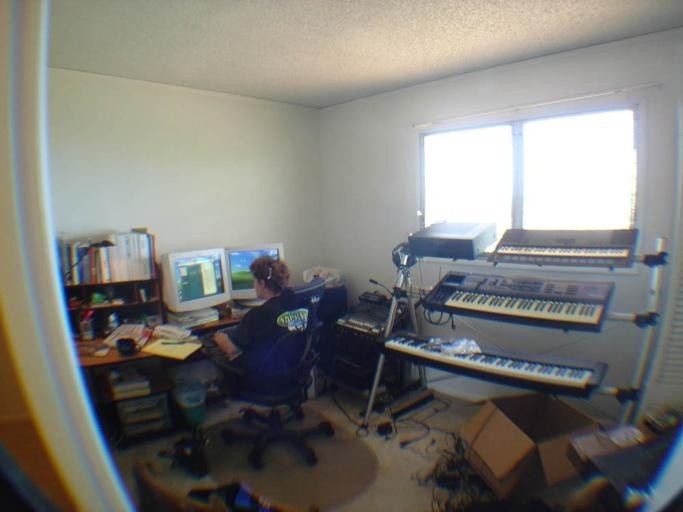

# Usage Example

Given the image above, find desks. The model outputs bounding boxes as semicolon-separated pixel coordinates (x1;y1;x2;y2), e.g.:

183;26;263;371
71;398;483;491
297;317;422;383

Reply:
189;317;249;332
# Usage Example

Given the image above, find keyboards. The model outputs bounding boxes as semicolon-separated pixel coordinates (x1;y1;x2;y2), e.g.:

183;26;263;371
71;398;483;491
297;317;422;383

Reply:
217;323;240;341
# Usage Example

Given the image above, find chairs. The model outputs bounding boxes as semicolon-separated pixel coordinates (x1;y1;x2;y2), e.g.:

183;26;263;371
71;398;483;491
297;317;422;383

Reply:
203;277;335;466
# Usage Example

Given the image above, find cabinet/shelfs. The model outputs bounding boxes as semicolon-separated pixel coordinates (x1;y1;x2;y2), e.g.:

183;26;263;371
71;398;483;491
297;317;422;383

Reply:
65;262;178;449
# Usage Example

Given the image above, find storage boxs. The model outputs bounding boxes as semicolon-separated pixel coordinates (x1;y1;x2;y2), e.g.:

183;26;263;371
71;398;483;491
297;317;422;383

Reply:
456;392;603;501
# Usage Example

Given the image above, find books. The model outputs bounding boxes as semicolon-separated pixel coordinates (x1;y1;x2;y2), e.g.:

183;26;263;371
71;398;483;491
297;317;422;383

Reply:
56;232;157;286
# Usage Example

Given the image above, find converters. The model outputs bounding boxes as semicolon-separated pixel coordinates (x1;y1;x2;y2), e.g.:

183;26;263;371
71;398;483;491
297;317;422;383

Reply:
377;422;392;435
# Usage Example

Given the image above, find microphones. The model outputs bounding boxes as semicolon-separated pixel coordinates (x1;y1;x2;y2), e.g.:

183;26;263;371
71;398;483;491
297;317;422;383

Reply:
369;279;404;314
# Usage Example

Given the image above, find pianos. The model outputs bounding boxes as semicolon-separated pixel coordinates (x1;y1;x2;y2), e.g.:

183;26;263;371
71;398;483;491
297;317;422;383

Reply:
421;271;615;332
383;330;605;398
490;230;638;268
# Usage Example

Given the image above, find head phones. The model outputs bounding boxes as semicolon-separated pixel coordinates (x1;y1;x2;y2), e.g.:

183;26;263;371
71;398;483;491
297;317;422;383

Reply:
392;243;416;268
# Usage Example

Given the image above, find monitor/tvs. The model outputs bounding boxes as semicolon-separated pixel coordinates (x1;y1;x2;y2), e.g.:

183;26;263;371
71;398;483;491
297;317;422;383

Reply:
157;248;231;318
224;243;285;307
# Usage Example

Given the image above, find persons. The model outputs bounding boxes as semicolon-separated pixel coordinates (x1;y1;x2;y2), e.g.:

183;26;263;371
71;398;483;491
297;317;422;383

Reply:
213;254;320;396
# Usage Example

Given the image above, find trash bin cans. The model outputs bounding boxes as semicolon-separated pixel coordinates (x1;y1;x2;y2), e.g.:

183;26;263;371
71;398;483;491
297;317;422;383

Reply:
172;382;206;426
